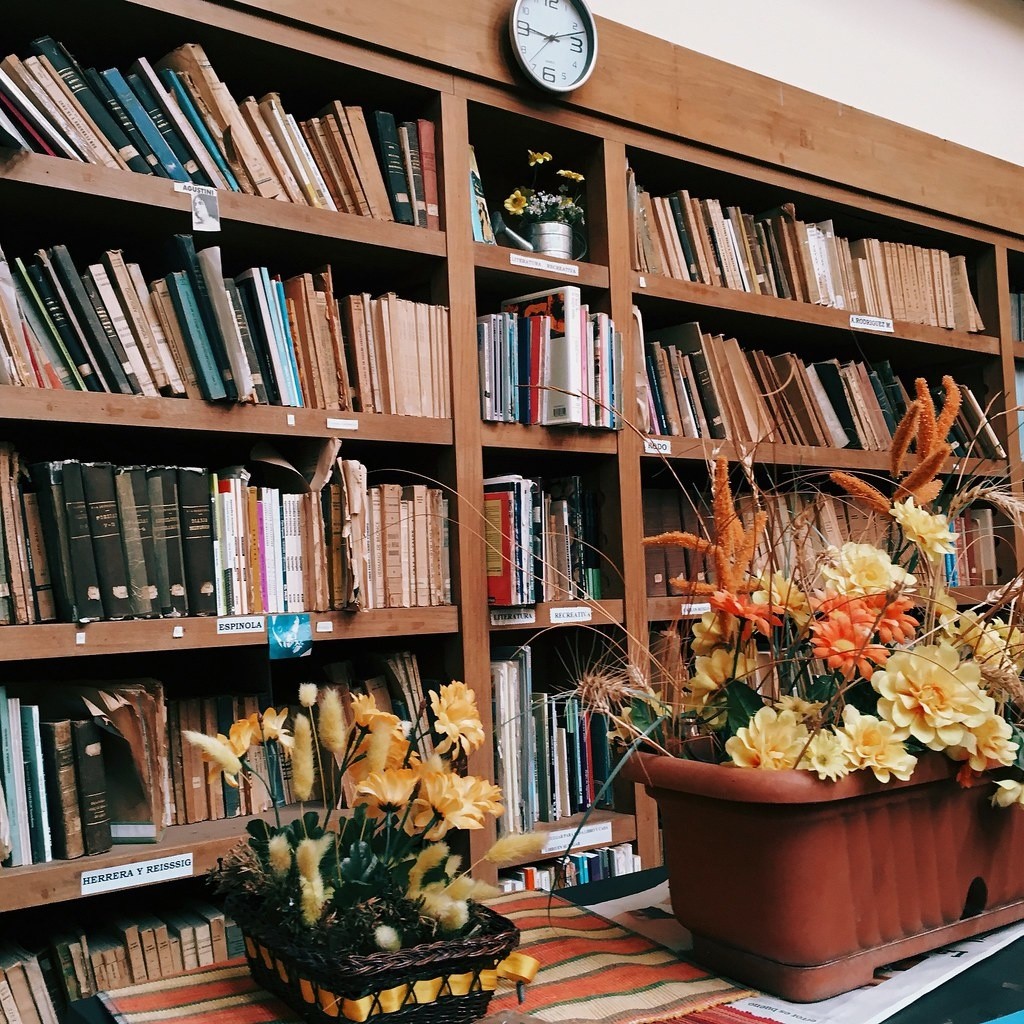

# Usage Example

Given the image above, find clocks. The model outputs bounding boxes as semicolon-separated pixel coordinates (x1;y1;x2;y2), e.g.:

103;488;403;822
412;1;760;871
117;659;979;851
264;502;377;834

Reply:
509;0;598;92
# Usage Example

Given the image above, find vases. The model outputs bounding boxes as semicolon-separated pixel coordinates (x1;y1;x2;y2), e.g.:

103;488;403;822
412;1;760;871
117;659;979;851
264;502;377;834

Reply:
616;749;1024;1005
525;222;573;260
208;889;539;1024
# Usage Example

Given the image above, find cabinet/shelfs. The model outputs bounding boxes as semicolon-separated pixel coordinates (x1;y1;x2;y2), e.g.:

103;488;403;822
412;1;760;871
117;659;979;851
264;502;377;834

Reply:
0;1;1024;1024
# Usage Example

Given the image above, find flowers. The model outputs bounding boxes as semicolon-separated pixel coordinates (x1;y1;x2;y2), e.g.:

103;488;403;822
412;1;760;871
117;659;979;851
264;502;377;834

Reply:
504;148;586;226
178;353;1024;951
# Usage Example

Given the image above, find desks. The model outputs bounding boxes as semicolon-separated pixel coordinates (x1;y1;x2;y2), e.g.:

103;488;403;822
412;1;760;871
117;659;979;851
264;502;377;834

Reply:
65;863;1024;1024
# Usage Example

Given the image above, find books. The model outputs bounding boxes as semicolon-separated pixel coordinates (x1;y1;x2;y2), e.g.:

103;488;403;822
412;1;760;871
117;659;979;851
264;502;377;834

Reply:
0;43;1024;1024
